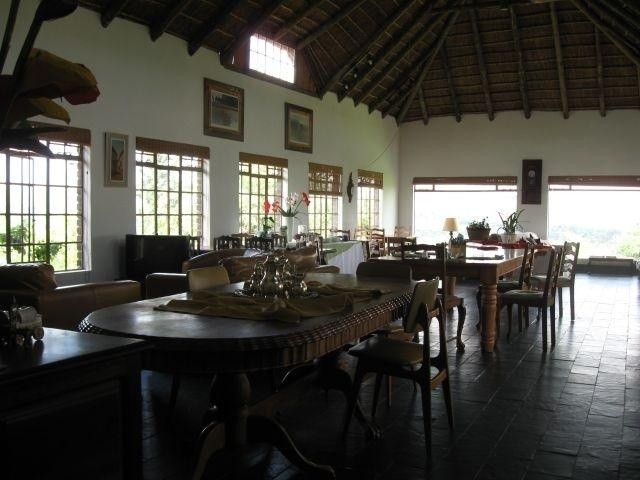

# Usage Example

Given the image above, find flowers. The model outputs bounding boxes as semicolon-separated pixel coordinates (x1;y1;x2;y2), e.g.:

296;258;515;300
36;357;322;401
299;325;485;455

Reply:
258;200;275;234
271;188;312;227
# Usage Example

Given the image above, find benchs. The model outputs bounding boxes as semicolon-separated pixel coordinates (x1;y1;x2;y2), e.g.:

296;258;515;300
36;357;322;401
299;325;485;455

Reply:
584;255;637;276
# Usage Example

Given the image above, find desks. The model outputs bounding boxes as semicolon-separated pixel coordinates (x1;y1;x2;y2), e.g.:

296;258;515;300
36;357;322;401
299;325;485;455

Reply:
77;273;425;479
368;247;548;351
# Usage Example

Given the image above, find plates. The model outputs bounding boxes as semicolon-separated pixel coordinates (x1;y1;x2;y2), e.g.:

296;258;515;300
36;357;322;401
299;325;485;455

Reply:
477;246;499;250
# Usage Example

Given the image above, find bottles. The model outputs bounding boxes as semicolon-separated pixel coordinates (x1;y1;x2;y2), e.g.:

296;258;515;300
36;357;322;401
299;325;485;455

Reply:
247;250;307;299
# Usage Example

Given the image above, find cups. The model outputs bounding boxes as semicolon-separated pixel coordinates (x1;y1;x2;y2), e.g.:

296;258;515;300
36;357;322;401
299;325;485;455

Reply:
255;225;320;250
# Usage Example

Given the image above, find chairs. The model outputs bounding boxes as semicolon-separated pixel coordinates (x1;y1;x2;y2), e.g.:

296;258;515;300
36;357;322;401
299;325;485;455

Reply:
475;246;538;341
493;248;566;353
2;237;416;331
537;242;579;322
337;281;455;461
400;241;466;351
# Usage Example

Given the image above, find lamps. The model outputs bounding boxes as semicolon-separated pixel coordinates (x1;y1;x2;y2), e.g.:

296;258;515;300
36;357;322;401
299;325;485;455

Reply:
442;217;462;239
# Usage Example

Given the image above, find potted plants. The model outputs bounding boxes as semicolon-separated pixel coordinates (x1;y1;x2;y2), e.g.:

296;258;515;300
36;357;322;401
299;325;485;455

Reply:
464;216;492;241
495;208;533;245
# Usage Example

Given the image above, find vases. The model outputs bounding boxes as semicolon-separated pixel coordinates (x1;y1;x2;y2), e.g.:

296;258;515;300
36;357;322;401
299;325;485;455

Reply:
202;76;247;143
103;130;130;190
282;216;295;243
282;100;315;155
